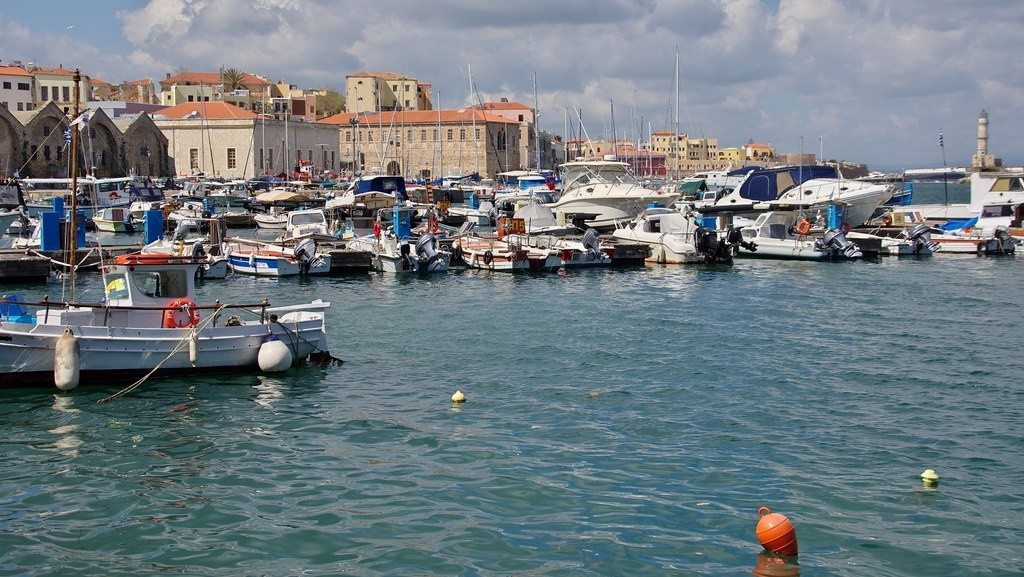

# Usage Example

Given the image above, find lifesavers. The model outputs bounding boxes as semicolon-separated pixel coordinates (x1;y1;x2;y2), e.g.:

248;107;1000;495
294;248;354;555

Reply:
884;215;892;225
164;299;201;329
432;218;438;232
373;223;381;237
483;250;492;265
840;222;850;235
109;192;118;201
454;245;462;260
797;221;810;235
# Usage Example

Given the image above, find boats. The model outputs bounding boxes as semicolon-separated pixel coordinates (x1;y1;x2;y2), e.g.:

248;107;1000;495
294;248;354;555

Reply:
0;67;332;377
0;45;1024;283
